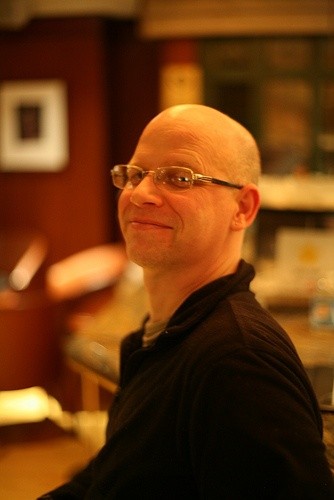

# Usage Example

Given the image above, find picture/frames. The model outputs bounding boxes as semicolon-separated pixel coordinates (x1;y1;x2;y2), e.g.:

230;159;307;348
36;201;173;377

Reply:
1;79;68;172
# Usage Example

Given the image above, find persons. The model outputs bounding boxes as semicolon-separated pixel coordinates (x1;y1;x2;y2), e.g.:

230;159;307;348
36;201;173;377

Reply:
35;101;333;500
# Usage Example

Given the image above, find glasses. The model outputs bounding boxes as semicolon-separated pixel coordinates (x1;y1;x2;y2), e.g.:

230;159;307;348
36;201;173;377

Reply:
111;164;244;193
